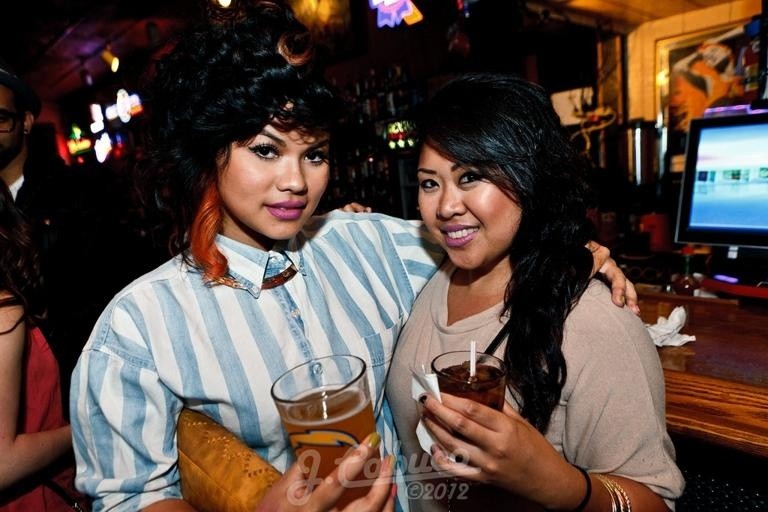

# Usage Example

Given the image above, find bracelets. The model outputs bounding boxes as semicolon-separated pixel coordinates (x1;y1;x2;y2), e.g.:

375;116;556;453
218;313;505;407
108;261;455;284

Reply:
596;472;634;511
568;460;592;511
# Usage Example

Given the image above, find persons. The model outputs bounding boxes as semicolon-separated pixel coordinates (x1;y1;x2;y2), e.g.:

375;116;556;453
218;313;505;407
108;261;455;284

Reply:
381;77;688;511
69;1;643;510
0;60;109;360
0;174;87;510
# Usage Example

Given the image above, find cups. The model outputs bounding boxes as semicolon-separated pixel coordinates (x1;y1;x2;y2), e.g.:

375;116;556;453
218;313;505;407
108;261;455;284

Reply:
271;354;389;510
430;349;509;462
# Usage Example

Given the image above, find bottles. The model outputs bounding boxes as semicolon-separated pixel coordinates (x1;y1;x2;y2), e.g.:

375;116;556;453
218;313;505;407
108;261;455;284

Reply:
672;247;698;296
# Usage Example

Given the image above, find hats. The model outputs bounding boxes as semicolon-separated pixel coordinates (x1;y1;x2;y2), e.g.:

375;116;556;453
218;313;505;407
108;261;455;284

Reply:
1;67;44;115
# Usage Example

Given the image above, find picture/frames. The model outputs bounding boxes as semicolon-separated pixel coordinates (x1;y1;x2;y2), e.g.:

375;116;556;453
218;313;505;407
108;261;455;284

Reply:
652;14;768;173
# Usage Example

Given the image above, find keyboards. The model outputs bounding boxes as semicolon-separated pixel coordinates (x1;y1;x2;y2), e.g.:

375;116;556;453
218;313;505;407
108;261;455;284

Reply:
677;464;768;512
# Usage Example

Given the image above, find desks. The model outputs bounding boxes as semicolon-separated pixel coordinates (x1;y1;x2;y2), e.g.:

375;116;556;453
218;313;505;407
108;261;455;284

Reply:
627;288;768;457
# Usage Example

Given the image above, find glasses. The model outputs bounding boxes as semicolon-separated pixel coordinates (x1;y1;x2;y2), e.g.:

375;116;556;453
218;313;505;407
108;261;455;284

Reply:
0;110;21;130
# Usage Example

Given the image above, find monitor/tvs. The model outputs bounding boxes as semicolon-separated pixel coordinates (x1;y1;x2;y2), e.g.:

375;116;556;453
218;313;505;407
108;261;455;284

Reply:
673;110;768;287
